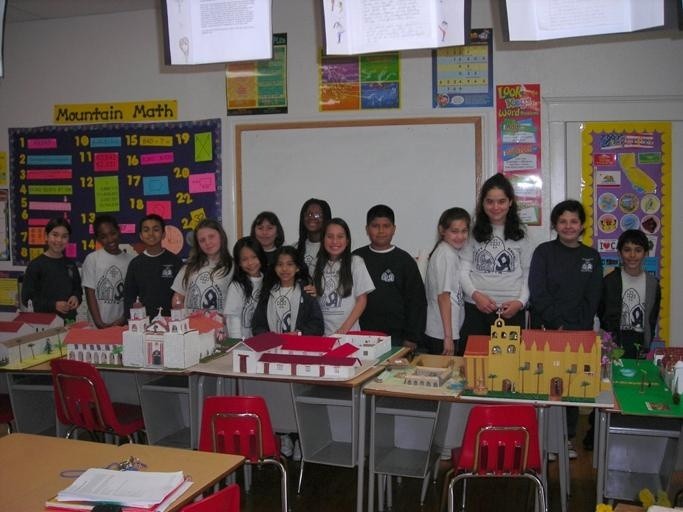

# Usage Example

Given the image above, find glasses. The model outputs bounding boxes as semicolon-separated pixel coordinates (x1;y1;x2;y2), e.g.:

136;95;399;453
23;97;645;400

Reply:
303;209;323;219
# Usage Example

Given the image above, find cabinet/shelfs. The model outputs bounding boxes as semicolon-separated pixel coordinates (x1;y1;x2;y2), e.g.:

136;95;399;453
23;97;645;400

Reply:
187;343;406;512
0;351;195;450
364;351;613;512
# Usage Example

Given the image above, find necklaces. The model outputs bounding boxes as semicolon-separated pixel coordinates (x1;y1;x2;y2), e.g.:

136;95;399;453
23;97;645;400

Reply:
327;260;336;270
278;287;292;302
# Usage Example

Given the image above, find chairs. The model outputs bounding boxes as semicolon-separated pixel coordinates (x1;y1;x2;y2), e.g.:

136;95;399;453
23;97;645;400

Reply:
183;481;240;512
198;395;289;512
50;359;143;446
448;402;547;512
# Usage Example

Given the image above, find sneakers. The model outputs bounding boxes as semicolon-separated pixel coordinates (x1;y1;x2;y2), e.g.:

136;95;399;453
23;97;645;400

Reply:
567;438;578;460
279;434;293;458
582;425;593;451
292;439;301;462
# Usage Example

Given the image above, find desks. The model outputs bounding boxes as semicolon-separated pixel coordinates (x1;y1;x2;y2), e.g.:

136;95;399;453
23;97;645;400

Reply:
0;432;244;512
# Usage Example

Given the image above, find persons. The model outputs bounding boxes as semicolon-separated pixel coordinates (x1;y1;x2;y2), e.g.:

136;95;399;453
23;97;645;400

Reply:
460;172;530;357
223;236;265;340
81;215;140;330
251;245;324;461
250;212;317;298
312;218;376;338
424;207;471;358
113;214;185;327
292;198;331;280
583;228;661;451
351;204;428;353
528;200;603;462
169;219;235;311
22;217;83;326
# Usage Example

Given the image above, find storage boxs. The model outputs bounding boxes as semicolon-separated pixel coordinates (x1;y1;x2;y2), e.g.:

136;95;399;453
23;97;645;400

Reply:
604;471;663;504
606;427;681;473
